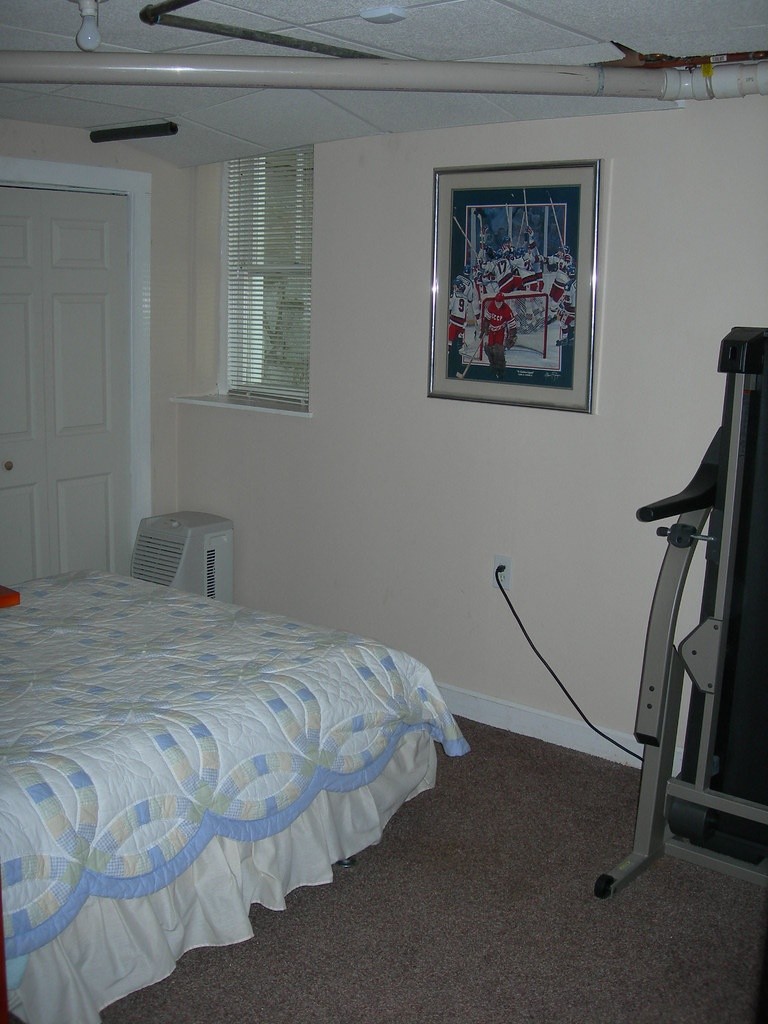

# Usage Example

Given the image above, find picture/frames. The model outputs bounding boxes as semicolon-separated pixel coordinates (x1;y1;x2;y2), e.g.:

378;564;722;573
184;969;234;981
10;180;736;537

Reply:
426;157;602;416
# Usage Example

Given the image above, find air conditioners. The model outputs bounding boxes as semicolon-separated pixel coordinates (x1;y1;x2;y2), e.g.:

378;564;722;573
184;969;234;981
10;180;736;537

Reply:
132;510;236;604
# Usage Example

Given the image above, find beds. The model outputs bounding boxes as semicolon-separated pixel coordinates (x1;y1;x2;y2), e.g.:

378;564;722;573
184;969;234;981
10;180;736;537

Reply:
0;570;471;1024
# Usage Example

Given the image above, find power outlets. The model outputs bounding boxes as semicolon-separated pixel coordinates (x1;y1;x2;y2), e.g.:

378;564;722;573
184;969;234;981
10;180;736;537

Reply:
493;554;512;591
